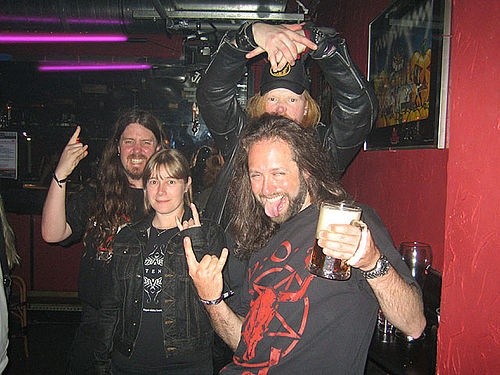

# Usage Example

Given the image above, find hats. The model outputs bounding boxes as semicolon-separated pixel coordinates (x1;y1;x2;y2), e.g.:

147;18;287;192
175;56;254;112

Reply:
259;59;312;97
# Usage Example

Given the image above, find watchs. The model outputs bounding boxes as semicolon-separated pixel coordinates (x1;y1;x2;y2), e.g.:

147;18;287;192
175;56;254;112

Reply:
359;255;391;280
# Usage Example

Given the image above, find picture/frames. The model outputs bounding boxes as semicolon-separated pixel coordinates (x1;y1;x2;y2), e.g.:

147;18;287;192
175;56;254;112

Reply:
363;0;454;151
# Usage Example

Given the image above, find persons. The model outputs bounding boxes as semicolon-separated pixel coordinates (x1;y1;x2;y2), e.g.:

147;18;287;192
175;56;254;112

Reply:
41;109;168;375
184;111;425;375
41;19;426;375
106;147;232;375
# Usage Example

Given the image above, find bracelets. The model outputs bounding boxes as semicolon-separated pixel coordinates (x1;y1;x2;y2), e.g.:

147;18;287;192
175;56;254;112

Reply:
52;172;67;188
197;290;234;306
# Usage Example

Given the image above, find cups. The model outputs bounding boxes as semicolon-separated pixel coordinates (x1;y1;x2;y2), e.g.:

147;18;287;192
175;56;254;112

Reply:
400;241;432;279
307;200;363;281
377;306;394;333
